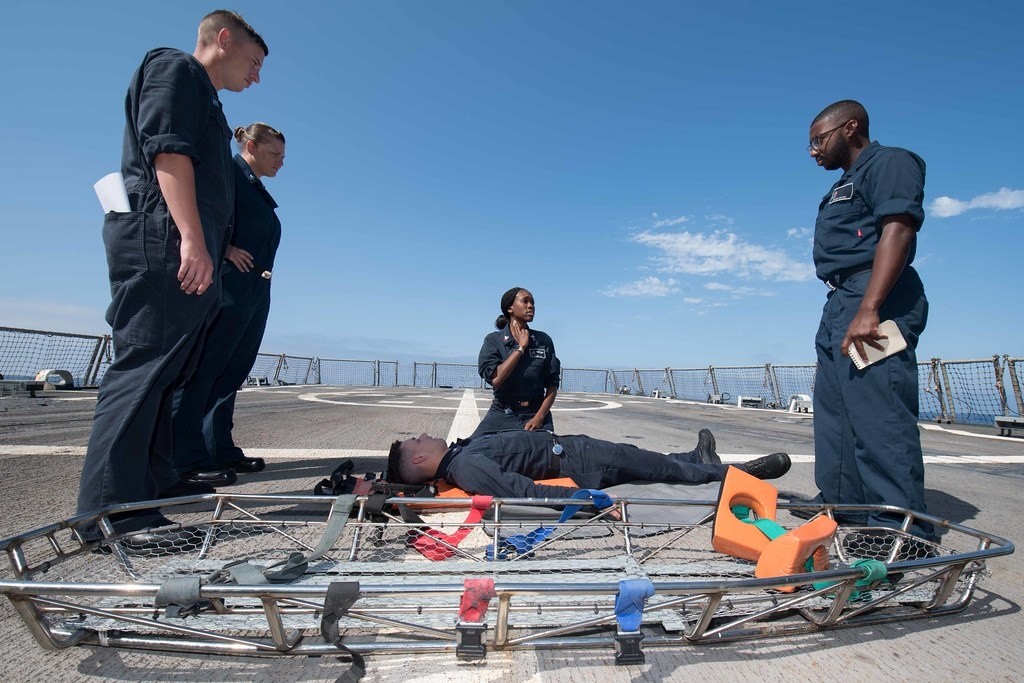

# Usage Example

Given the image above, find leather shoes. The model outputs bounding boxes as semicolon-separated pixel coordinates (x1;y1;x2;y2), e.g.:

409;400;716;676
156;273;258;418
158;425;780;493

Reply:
188;466;237;486
233;457;265;472
157;479;216;505
86;516;205;556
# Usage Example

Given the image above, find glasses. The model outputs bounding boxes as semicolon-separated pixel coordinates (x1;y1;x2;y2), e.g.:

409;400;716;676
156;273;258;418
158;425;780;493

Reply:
807;122;847;151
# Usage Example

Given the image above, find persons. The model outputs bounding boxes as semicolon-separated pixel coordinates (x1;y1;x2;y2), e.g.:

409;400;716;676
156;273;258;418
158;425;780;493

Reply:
470;287;561;436
171;122;285;487
71;10;268;557
386;430;792;520
789;100;936;561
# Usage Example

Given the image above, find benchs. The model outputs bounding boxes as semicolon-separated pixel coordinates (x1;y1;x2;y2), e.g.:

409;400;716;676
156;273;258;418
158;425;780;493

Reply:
0;379;46;398
995;416;1024;436
247;376;271;387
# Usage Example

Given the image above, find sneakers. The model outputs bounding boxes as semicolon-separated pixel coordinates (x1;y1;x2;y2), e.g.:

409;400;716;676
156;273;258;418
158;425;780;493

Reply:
690;429;722;465
742;453;791;480
843;532;932;561
790;492;867;527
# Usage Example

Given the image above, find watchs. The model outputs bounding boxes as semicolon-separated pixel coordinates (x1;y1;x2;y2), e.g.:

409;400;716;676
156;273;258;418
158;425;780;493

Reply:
515;345;524;354
